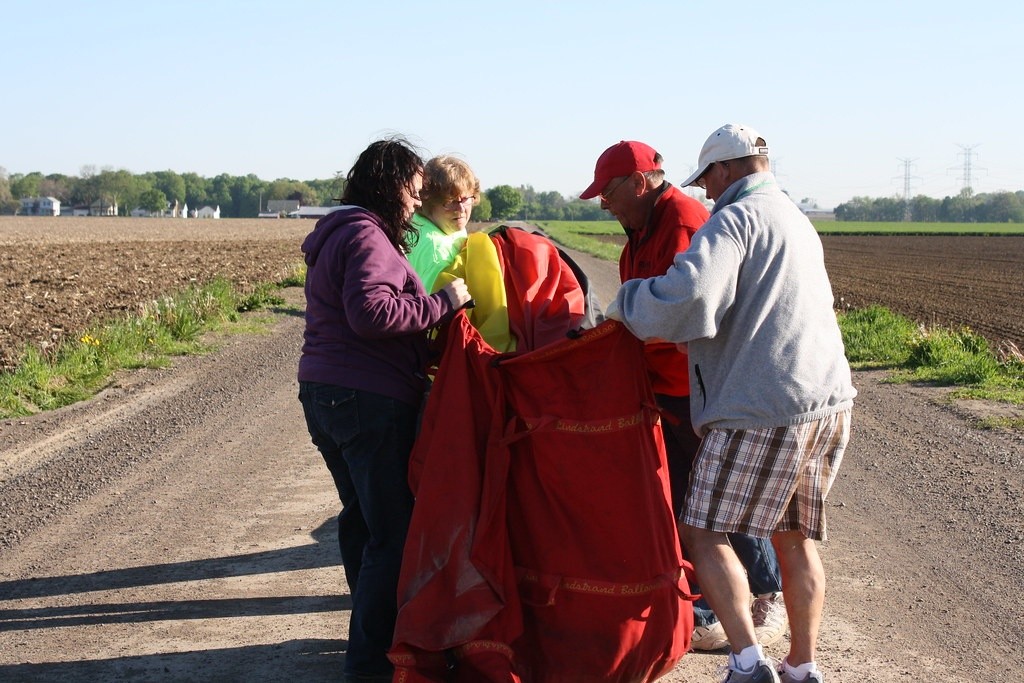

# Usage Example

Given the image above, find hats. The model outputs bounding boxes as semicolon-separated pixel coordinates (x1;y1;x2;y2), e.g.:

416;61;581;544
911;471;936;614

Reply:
681;124;768;188
579;141;661;200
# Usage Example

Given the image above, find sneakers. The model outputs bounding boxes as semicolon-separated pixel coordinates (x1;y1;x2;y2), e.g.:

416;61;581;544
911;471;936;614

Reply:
766;654;823;683
750;591;789;648
711;652;783;683
690;621;729;652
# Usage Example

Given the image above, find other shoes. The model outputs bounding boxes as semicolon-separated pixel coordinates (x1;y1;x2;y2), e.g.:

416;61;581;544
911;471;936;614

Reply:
345;671;393;683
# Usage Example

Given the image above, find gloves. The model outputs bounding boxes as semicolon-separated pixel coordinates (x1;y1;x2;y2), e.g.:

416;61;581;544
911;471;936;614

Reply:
605;299;624;322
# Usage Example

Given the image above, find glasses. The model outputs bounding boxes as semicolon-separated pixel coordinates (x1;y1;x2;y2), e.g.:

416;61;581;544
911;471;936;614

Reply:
695;160;729;189
430;196;476;211
600;172;649;206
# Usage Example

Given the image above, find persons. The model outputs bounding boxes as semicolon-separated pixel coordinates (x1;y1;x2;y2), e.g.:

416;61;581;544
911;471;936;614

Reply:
400;154;478;296
298;140;471;683
605;124;857;683
581;141;788;651
